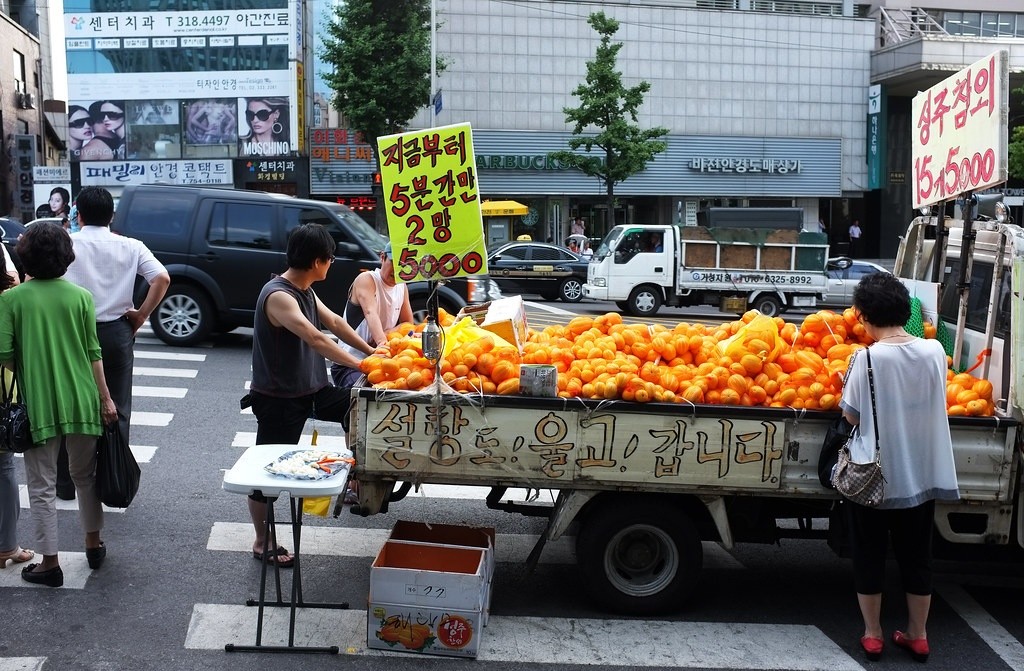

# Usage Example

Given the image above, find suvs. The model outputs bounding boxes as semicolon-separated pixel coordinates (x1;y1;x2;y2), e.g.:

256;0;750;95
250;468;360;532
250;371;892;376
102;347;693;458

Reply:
111;185;504;346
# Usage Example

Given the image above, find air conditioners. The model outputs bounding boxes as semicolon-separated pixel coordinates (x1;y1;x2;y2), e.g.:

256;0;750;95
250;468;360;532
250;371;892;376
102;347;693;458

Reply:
26;94;35;109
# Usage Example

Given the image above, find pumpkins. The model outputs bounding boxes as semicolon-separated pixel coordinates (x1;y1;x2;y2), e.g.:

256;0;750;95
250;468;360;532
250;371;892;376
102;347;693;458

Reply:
360;309;994;418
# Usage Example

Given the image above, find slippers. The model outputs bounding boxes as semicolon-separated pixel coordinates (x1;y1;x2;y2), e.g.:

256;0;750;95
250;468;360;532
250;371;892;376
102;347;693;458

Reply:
253;546;294;569
343;490;360;504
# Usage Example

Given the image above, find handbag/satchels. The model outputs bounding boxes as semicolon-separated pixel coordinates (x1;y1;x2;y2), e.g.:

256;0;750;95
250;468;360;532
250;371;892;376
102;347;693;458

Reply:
830;437;888;507
95;418;141;509
818;417;855;489
0;359;38;453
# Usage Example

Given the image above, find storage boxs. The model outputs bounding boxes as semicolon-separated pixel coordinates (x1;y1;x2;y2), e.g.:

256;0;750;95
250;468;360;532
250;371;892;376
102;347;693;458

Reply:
366;520;496;657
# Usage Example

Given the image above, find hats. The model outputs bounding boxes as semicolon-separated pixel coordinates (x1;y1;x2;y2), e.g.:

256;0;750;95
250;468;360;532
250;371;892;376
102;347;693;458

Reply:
384;240;393;261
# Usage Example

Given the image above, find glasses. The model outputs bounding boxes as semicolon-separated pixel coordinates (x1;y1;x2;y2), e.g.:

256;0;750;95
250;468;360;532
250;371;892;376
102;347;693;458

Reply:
246;108;277;121
328;254;336;264
99;112;124;121
856;312;864;325
69;117;95;129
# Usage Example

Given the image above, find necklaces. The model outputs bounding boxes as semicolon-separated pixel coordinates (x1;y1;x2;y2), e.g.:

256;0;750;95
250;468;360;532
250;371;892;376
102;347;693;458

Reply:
879;334;911;340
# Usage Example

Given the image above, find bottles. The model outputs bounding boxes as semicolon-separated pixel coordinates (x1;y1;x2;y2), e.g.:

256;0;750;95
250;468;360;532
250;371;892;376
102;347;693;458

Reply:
422;316;441;359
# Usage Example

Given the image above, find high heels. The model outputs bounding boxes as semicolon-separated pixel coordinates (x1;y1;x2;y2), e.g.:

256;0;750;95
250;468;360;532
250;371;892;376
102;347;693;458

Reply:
0;545;34;569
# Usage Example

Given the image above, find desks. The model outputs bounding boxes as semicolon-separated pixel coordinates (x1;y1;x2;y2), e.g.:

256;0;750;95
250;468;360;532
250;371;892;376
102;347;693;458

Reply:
223;444;353;656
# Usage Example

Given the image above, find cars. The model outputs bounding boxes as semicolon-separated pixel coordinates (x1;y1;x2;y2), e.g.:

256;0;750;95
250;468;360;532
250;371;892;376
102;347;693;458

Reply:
818;256;893;309
486;235;591;303
1;218;33;283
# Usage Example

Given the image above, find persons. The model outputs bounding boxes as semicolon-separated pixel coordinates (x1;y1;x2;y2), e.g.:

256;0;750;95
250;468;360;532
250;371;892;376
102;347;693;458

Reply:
849;221;863;257
183;100;237;144
825;271;960;656
333;241;414;388
650;233;662;252
58;186;170;501
247;98;290;157
819;219;826;232
565;216;594;259
0;218;118;587
240;223;386;567
68;101;125;160
0;237;35;568
48;188;70;218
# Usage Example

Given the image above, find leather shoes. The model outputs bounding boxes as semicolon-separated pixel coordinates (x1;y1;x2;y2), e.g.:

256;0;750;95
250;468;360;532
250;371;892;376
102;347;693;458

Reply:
86;540;106;570
22;562;64;587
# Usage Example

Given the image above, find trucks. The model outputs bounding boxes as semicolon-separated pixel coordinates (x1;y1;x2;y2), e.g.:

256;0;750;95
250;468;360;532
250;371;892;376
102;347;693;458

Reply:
347;203;1023;613
582;208;830;318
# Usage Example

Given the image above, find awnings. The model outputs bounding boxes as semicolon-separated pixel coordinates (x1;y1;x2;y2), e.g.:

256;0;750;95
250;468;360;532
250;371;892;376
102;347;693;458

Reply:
481;200;529;216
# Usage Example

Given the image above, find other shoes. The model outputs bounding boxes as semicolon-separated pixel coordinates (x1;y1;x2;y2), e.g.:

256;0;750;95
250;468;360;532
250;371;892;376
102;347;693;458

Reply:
859;634;885;661
892;629;930;663
